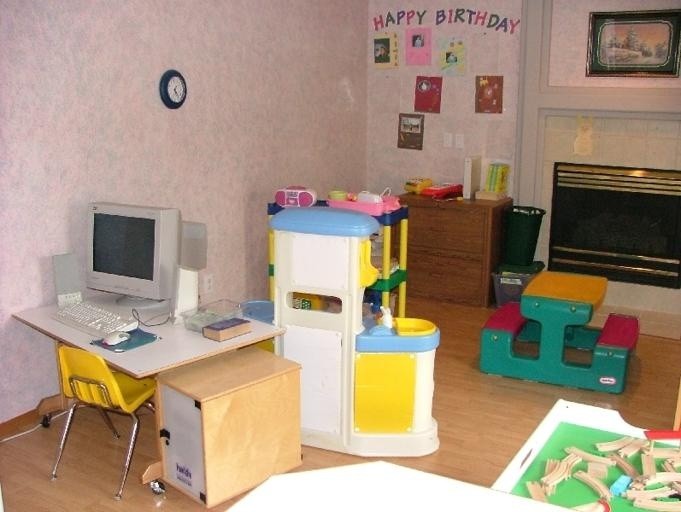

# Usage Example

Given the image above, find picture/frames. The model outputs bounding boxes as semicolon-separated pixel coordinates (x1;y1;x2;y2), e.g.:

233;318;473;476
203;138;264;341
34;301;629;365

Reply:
584;8;681;79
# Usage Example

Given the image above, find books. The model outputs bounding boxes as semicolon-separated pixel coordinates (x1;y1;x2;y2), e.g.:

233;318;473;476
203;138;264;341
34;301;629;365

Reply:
485;163;510;193
202;318;252;342
462;155;481;200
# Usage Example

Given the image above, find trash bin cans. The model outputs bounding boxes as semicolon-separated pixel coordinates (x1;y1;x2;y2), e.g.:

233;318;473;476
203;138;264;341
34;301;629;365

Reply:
504;206;546;265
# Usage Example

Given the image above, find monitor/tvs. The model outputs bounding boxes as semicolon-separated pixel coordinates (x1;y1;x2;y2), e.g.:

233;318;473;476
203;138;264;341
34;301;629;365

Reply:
87;201;208;310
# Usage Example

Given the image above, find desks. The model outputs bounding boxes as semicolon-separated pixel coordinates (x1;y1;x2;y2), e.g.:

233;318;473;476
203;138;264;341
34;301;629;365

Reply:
518;270;608;392
10;291;285;486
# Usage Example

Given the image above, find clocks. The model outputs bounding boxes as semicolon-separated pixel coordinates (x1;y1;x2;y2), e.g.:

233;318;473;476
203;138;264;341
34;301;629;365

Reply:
159;69;187;109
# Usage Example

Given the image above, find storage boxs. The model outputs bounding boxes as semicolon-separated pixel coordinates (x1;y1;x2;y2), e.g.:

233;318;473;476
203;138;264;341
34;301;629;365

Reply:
492;271;537;309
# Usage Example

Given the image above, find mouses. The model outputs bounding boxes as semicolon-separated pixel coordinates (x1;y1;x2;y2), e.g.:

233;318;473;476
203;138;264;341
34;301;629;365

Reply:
101;330;132;348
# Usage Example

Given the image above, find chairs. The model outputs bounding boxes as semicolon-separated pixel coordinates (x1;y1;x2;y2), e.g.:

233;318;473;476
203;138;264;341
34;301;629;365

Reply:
49;344;157;501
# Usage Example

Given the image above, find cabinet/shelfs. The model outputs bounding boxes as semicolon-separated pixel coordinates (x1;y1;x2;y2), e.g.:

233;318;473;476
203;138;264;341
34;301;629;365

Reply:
390;191;514;308
155;345;304;510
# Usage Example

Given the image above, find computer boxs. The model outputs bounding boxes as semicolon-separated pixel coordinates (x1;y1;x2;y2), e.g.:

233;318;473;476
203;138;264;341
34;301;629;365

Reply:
157;387;207;505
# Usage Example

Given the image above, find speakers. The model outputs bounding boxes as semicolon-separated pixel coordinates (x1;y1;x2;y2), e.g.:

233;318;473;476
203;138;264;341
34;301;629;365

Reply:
170;261;199;328
48;251;84;308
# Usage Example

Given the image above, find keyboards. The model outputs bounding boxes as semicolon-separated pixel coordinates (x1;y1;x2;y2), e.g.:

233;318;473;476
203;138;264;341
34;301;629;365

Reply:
50;300;138;338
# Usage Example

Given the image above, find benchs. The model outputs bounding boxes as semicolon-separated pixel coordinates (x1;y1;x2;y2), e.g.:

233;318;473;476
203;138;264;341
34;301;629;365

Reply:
590;313;640;395
479;300;529;381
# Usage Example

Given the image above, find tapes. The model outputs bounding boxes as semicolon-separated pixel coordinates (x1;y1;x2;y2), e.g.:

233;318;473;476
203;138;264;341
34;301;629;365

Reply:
328;191;348;200
